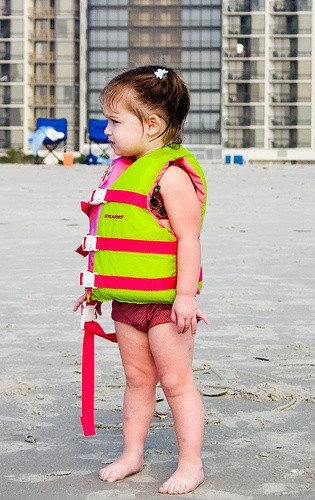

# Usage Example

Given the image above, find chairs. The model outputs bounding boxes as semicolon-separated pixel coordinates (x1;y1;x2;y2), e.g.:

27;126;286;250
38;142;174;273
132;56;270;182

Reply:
88;119;110;158
34;118;67;165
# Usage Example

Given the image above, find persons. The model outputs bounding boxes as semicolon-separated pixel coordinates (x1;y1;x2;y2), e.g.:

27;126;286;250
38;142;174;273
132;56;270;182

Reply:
73;65;210;494
